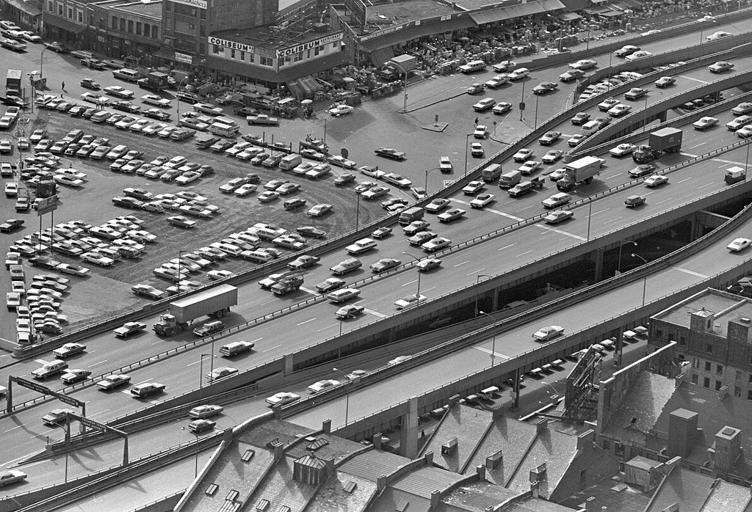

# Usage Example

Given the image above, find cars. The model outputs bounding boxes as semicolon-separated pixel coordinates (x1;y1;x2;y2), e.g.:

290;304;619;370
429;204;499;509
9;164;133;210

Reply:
0;467;30;487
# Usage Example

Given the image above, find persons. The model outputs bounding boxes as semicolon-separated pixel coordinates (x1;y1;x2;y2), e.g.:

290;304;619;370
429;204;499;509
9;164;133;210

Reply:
473;117;479;127
60;92;64;98
95;98;99;109
102;100;105;110
61;81;65;89
